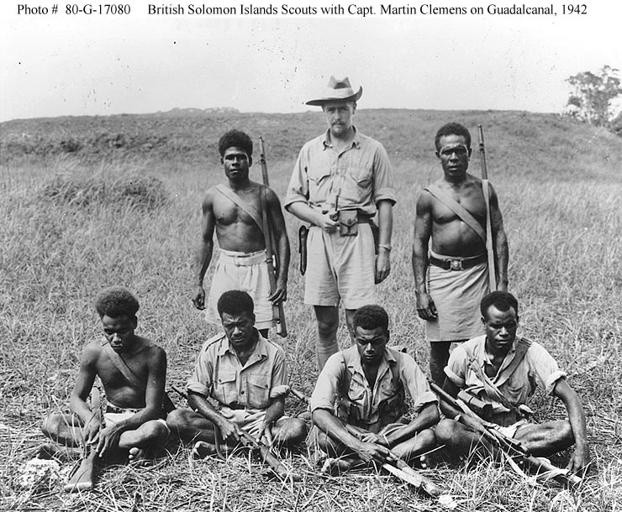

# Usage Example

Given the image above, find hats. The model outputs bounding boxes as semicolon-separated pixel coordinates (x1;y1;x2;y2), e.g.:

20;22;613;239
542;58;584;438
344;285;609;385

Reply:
306;76;364;105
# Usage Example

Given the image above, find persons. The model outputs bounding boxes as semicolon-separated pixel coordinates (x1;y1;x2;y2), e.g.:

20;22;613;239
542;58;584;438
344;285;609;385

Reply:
168;291;308;458
190;128;291;340
433;288;595;475
410;122;510;390
38;283;175;466
282;75;399;374
304;305;441;473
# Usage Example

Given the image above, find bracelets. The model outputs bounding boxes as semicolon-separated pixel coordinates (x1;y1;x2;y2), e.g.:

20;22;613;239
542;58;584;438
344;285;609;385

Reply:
378;243;392;252
498;276;509;287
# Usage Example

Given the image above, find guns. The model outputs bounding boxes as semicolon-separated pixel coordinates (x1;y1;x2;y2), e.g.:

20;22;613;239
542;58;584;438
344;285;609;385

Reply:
64;381;101;491
478;124;488;178
258;137;287;337
429;379;582;489
290;388;441;497
169;382;300;481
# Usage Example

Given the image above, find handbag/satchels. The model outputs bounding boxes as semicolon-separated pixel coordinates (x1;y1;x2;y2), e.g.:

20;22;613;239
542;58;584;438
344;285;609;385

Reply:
298;225;307;276
369;219;380;255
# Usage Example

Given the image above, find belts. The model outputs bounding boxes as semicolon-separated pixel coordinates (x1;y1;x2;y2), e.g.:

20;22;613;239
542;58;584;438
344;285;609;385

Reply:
223;402;244;410
106;406;139;414
428;254;487;271
311;213;370;227
221;253;266;266
